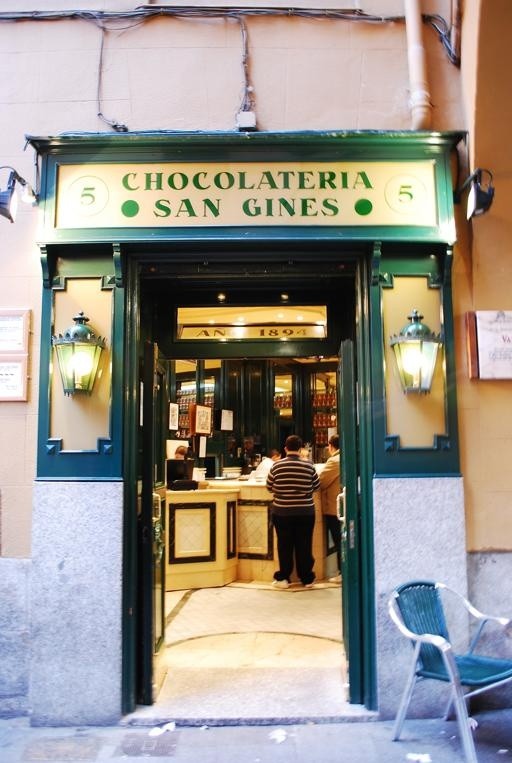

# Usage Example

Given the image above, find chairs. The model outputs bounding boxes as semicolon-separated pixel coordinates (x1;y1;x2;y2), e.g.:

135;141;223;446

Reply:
386;578;512;763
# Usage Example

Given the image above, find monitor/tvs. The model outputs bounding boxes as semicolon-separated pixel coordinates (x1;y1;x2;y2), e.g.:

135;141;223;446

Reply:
201;456;216;478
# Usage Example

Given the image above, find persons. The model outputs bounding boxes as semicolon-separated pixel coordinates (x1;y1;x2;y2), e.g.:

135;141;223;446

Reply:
315;434;342;584
175;446;187;459
243;437;256;457
254;448;280;478
267;436;321;585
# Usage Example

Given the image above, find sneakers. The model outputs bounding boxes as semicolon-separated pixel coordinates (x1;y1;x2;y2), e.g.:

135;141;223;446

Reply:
271;578;292;590
305;583;314;589
328;573;343;584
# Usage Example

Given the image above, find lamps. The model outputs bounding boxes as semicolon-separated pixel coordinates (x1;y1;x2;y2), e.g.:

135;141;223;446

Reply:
0;165;38;224
453;166;495;221
387;308;444;397
50;309;108;398
